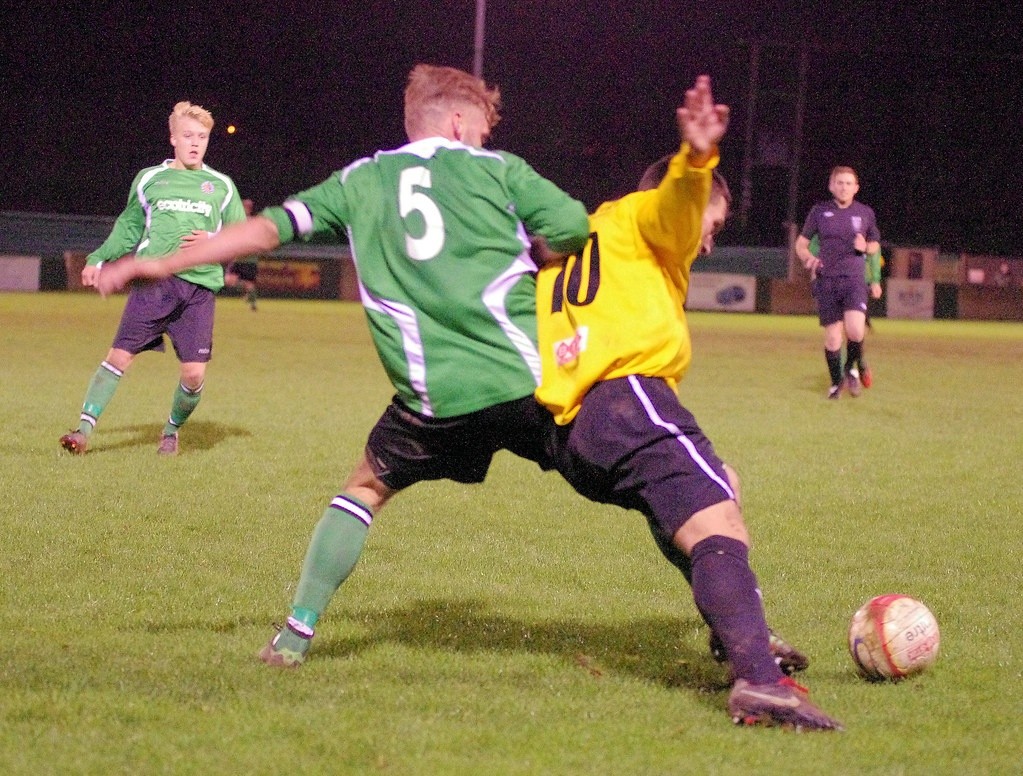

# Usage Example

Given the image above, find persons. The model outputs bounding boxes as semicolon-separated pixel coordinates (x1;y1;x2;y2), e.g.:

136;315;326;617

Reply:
95;63;730;661
810;235;882;388
59;99;248;456
532;70;840;730
795;165;880;397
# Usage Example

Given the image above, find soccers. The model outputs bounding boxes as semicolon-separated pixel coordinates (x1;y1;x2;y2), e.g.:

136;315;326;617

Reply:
847;593;941;682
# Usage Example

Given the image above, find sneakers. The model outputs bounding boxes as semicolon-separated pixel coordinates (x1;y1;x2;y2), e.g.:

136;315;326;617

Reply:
726;676;844;732
258;621;301;670
828;377;845;399
708;626;810;675
157;430;179;455
847;368;861;398
60;428;87;454
861;367;871;388
